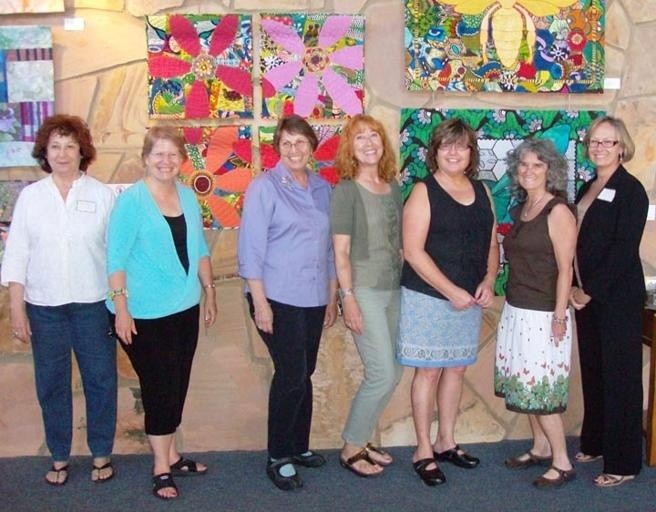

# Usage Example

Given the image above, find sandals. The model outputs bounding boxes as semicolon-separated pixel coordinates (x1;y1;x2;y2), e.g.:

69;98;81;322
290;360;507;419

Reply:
532;464;577;493
507;451;554;470
290;448;328;469
572;451;604;463
593;474;636;490
165;456;209;477
264;457;301;493
150;472;179;502
431;445;481;470
410;457;447;489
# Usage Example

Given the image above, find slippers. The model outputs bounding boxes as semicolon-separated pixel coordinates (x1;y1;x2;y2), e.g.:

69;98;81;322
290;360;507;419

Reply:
43;462;70;489
88;460;115;486
337;442;385;477
363;441;395;466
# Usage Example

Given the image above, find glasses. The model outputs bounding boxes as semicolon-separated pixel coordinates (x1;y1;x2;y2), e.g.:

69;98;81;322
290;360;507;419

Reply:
437;140;471;153
585;139;622;149
275;139;314;149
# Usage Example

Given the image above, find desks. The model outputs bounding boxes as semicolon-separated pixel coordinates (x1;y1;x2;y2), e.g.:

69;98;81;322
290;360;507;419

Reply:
642;309;656;467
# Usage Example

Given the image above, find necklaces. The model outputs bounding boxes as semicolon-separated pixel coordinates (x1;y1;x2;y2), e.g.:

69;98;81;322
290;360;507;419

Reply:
520;200;540;218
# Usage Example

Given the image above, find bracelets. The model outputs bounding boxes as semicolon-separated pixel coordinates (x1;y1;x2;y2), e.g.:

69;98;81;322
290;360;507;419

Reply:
340;289;353;299
106;289;128;298
552;315;570;324
205;285;217;291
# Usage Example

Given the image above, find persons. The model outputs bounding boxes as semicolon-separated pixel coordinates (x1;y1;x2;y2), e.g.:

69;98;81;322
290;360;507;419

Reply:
1;113;113;487
566;116;646;487
329;114;401;478
495;139;577;490
400;118;500;487
105;127;218;495
236;115;339;489
